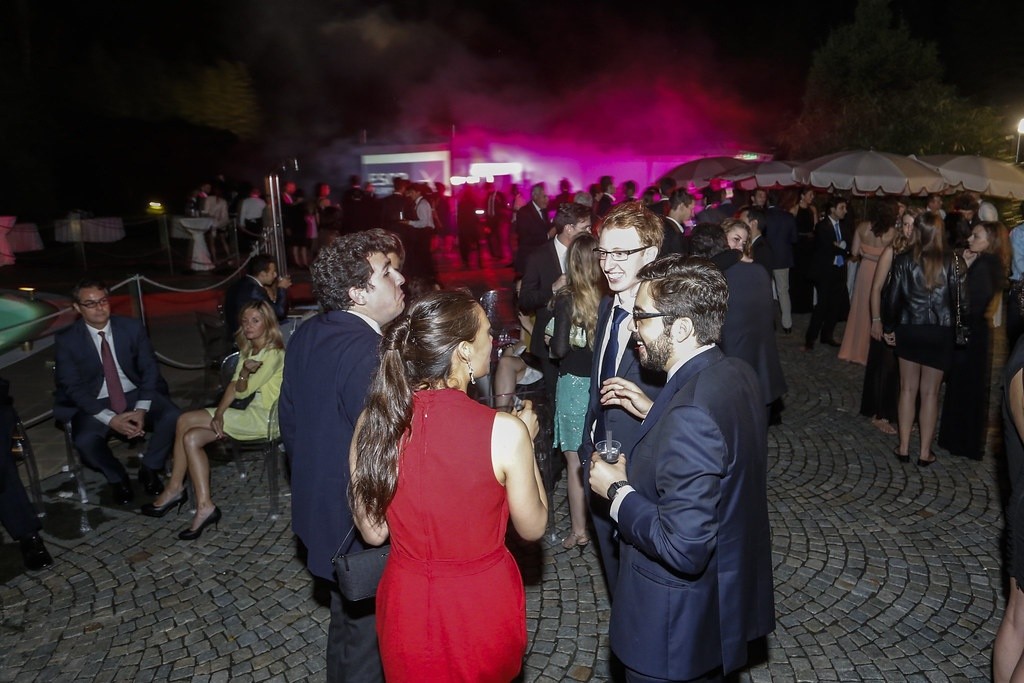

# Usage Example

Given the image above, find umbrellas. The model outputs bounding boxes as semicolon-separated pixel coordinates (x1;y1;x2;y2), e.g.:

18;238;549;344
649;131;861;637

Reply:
657;146;1024;222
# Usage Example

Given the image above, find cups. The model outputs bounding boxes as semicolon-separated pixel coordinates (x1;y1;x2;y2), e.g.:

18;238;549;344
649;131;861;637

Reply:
595;440;622;464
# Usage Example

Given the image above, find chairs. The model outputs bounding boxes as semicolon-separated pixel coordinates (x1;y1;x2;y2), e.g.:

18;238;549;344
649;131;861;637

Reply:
0;302;281;520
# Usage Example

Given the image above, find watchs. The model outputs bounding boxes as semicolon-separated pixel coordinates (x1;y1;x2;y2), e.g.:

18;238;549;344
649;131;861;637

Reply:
607;481;632;500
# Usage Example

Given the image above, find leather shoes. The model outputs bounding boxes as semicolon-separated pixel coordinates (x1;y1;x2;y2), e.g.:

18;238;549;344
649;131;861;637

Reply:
139;469;164;495
114;479;135;504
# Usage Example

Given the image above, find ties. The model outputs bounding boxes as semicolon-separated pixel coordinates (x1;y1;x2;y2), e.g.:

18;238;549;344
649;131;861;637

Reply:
97;331;127;413
835;223;843;268
593;306;628;450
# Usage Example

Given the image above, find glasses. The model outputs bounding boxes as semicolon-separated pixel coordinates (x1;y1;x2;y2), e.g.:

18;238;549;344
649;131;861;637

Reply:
593;244;656;261
633;310;674;330
79;298;109;308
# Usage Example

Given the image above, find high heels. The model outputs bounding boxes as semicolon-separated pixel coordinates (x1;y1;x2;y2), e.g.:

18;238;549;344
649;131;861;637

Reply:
562;532;589;556
179;503;222;540
142;487;189;518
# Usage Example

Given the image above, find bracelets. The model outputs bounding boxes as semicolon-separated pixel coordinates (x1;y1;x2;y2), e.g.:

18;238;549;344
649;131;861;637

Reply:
872;317;881;321
239;376;249;381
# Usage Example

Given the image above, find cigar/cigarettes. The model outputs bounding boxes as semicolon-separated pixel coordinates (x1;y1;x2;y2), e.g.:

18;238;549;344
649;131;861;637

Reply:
216;433;219;437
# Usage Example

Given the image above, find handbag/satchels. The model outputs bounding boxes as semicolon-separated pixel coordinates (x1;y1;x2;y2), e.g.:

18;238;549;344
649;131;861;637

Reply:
955;324;973;345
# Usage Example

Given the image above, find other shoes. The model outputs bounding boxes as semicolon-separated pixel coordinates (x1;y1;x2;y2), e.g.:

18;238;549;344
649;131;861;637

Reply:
783;328;791;334
918;451;937;466
20;535;53;570
894;445;910;463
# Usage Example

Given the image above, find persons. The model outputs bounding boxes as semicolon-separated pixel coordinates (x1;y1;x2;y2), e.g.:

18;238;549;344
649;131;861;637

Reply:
0;377;53;571
186;174;452;290
798;195;1024;683
223;252;293;343
141;297;286;541
277;201;789;682
456;176;819;334
53;277;183;506
